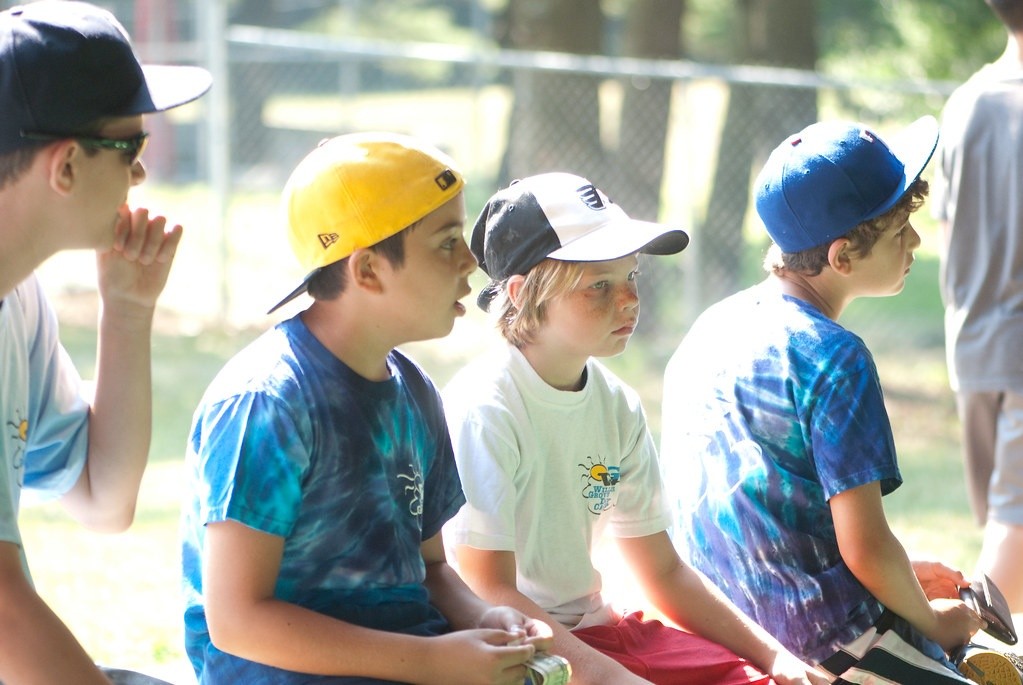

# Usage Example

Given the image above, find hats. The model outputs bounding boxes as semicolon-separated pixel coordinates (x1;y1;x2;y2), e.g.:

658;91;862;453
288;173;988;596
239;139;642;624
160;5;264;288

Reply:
469;173;690;316
1;0;214;144
753;112;940;253
261;127;466;315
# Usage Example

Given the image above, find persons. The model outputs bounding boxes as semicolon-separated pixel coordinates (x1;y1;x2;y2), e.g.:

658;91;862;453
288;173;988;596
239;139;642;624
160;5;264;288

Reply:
656;112;1023;684
437;170;836;684
178;132;553;684
1;0;213;685
934;1;1023;668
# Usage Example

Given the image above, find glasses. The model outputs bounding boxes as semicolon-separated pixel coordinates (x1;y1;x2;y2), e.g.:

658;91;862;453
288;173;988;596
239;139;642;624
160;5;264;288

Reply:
18;132;149;166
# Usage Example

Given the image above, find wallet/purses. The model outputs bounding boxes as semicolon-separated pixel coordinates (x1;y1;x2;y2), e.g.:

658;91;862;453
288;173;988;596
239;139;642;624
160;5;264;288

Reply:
957;572;1017;646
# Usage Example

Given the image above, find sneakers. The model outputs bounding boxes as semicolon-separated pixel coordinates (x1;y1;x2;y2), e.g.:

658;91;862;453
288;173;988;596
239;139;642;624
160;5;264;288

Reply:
952;650;1023;685
